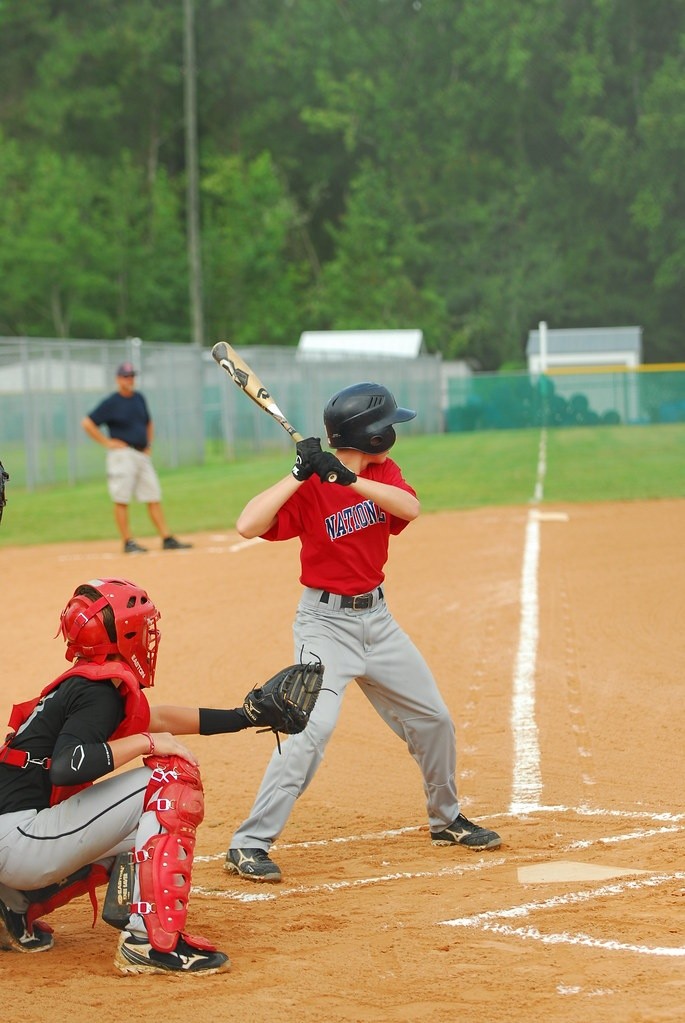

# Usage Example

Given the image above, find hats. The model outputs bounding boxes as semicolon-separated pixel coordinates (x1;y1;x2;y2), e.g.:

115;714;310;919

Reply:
117;363;135;376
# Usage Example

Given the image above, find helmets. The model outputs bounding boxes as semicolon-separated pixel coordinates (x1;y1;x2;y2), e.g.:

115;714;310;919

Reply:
323;383;417;455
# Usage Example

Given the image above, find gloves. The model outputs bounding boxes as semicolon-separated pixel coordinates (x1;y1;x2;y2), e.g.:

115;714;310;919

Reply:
309;452;355;486
291;437;322;481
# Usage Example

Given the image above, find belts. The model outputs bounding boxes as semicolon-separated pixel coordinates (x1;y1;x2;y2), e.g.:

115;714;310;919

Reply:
320;587;383;611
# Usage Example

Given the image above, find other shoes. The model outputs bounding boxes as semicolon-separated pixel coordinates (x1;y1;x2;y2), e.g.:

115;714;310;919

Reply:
163;536;192;549
123;540;148;553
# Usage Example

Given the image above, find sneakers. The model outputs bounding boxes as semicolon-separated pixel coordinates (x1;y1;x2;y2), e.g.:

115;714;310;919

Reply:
113;928;232;978
223;846;281;883
0;900;54;953
431;812;502;852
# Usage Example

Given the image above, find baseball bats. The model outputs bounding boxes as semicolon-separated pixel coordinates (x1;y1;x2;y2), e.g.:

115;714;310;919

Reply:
210;340;339;482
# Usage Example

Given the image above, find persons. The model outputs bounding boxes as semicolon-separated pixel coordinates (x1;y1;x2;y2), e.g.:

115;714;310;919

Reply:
82;361;194;553
0;577;279;978
223;382;502;884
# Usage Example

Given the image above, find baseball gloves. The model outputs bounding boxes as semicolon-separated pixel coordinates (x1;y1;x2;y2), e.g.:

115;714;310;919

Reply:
241;661;323;735
